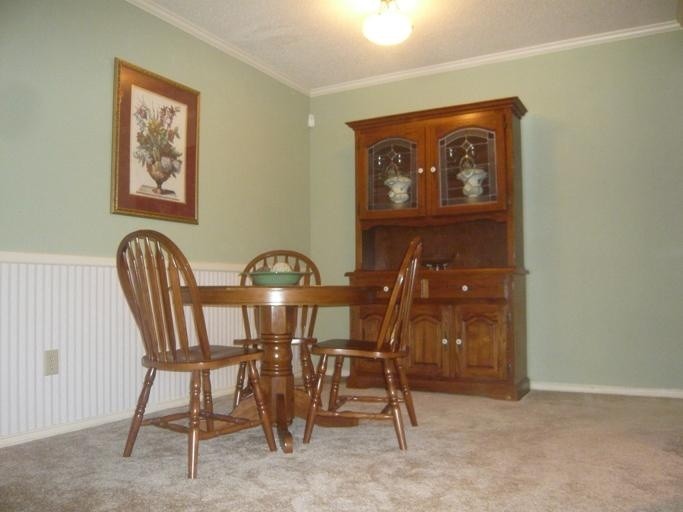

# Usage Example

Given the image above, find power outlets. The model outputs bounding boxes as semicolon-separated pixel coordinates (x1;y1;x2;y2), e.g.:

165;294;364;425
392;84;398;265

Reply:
44;348;58;374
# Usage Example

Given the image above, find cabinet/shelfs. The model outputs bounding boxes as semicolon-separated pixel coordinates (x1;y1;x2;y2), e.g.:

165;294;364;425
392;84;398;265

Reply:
344;96;531;400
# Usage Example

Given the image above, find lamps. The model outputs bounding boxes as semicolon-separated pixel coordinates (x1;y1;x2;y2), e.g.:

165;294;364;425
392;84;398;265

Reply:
359;0;415;47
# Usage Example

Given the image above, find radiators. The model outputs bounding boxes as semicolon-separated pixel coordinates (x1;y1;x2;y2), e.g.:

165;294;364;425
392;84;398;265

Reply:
1;251;305;448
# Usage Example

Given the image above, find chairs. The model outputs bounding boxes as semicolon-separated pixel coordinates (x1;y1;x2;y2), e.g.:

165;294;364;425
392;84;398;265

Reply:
116;230;277;478
233;250;321;410
303;236;424;452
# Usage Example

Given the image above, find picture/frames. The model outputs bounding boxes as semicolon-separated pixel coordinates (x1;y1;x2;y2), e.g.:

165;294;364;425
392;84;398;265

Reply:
111;57;202;226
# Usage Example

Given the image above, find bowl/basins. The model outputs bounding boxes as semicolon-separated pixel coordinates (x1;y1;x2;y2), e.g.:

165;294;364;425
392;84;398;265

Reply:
239;271;309;286
418;253;456;266
